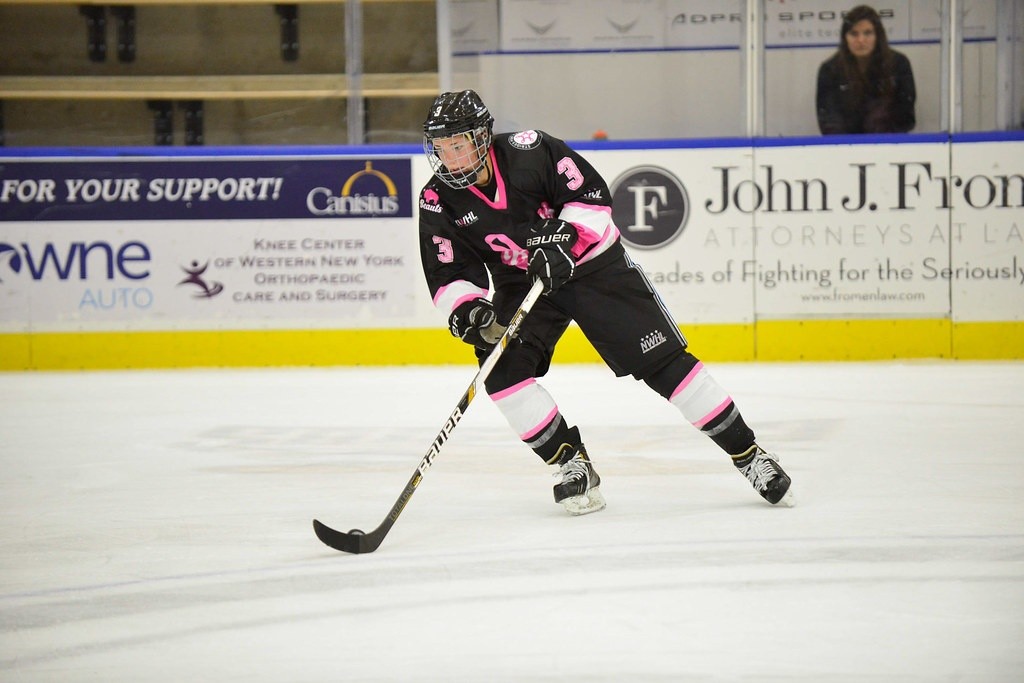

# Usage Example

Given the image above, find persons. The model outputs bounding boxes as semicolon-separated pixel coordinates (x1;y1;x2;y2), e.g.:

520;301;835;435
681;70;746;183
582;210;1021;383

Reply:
418;91;796;515
815;6;917;136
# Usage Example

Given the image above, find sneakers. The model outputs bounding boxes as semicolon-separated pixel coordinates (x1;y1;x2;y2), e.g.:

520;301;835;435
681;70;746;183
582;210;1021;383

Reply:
546;426;606;516
730;441;796;508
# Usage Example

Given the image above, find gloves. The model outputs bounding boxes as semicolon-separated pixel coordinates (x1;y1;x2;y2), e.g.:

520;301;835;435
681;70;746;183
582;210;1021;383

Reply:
448;297;520;354
526;218;577;298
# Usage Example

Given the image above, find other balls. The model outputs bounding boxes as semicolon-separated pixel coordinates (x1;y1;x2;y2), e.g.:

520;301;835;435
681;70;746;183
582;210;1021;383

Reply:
347;529;366;536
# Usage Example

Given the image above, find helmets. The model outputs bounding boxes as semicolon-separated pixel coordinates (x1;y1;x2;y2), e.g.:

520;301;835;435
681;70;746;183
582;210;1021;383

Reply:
422;90;495;190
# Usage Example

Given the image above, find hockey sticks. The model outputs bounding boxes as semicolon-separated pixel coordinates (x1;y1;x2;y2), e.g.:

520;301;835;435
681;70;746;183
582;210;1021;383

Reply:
313;279;544;555
